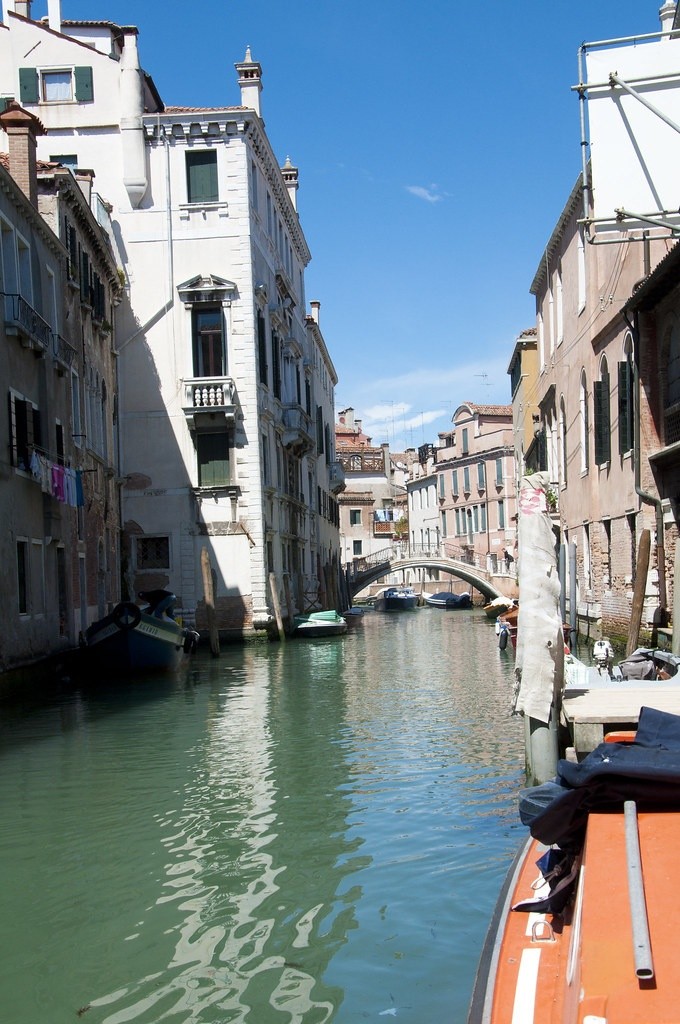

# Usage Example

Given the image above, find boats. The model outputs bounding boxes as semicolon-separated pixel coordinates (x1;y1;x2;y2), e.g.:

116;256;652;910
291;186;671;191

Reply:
483;596;514;621
500;604;574;651
422;589;470;608
341;607;364;618
297;609;348;638
81;600;200;692
466;731;680;1023
366;585;421;611
